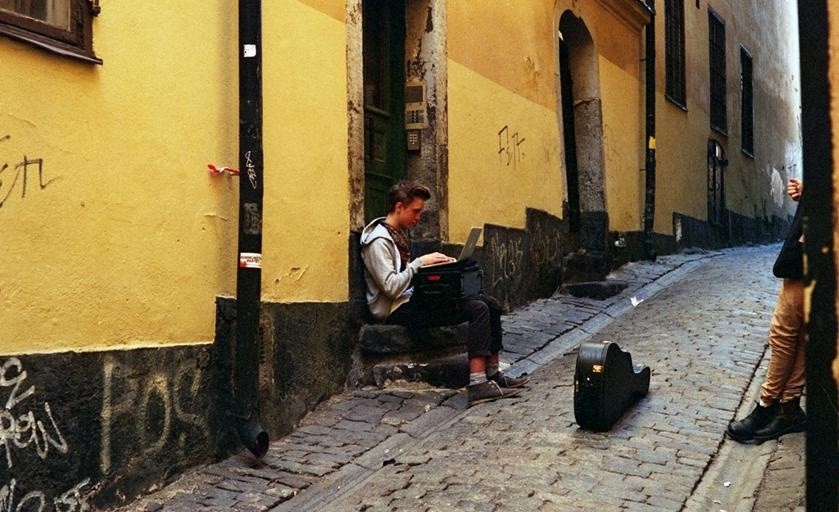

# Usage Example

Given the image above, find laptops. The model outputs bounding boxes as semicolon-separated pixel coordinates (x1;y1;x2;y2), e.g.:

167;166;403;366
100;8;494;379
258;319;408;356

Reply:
420;227;482;269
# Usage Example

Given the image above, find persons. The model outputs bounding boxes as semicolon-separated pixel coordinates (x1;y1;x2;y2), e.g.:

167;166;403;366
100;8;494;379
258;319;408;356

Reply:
726;177;806;445
359;178;530;407
